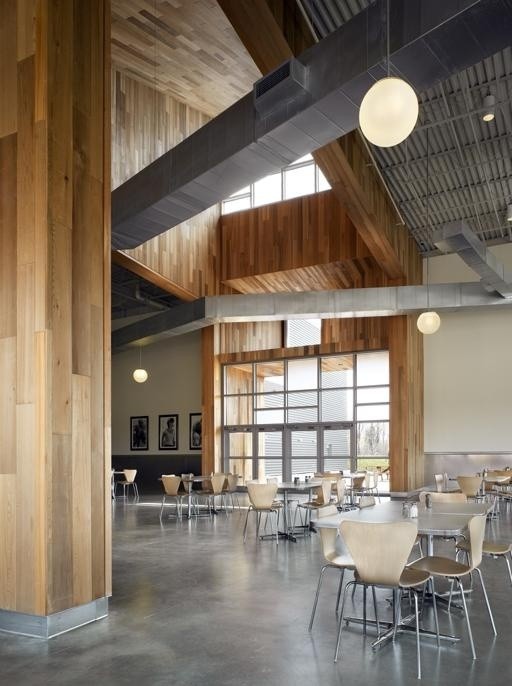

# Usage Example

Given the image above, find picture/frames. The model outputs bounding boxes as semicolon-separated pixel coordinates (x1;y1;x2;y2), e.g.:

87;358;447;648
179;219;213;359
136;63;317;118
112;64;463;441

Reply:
188;412;202;450
157;414;179;451
129;415;149;451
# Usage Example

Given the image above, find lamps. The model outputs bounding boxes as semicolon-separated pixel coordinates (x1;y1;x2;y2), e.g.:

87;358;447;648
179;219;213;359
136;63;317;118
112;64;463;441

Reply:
133;345;149;384
507;203;512;221
483;94;495;122
357;0;420;148
416;118;442;336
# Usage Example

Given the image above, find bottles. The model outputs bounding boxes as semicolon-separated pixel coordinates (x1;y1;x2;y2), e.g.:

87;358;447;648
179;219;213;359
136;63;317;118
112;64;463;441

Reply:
425;494;432;508
402;499;418;519
293;475;312;485
